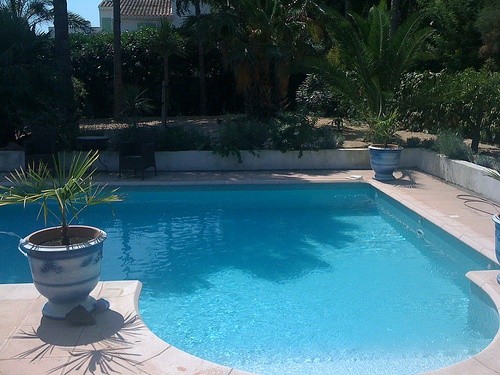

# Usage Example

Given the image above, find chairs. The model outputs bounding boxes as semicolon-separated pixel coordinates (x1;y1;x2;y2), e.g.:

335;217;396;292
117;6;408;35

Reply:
119;129;157;180
24;128;58;178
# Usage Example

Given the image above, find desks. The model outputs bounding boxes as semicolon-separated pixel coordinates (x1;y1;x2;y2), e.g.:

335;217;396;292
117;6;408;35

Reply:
76;136;110;174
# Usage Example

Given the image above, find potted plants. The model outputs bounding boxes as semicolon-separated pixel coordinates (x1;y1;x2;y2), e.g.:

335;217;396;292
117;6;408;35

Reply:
0;149;122;320
366;106;404;181
